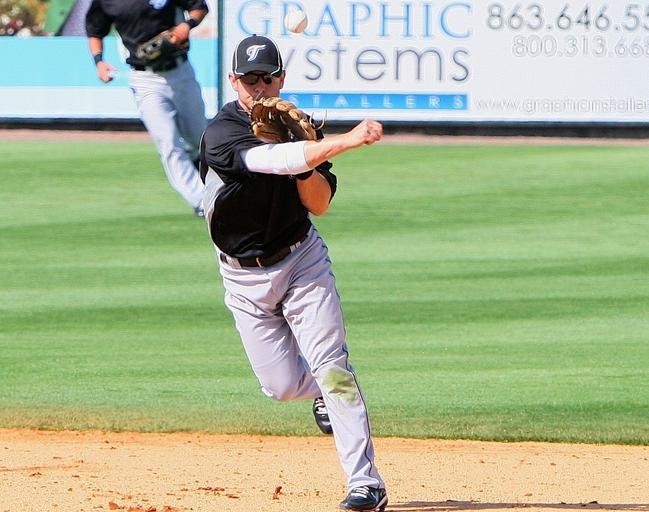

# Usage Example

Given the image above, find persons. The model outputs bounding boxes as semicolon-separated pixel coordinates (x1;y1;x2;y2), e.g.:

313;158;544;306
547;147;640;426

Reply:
84;1;210;218
195;30;389;511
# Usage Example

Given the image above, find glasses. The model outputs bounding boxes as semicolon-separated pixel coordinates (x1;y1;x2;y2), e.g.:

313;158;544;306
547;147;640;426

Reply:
240;71;272;85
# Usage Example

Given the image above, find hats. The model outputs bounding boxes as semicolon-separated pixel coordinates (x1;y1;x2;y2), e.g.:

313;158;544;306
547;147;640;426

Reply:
231;33;282;79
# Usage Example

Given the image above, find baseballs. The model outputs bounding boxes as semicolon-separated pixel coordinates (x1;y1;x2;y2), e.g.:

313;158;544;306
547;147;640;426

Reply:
286;10;307;33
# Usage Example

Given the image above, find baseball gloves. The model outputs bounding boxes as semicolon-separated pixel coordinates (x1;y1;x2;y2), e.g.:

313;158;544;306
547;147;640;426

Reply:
250;98;316;143
137;32;180;61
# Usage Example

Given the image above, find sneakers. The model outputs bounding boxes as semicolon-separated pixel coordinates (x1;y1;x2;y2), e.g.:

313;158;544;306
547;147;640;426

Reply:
339;485;389;512
312;396;334;435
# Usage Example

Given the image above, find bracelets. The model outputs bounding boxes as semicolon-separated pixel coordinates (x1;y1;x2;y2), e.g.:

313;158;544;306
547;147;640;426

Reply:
93;52;102;64
183;18;196;29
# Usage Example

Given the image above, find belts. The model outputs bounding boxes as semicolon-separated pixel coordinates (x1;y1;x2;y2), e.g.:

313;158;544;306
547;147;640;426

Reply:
211;222;315;268
130;54;188;71
